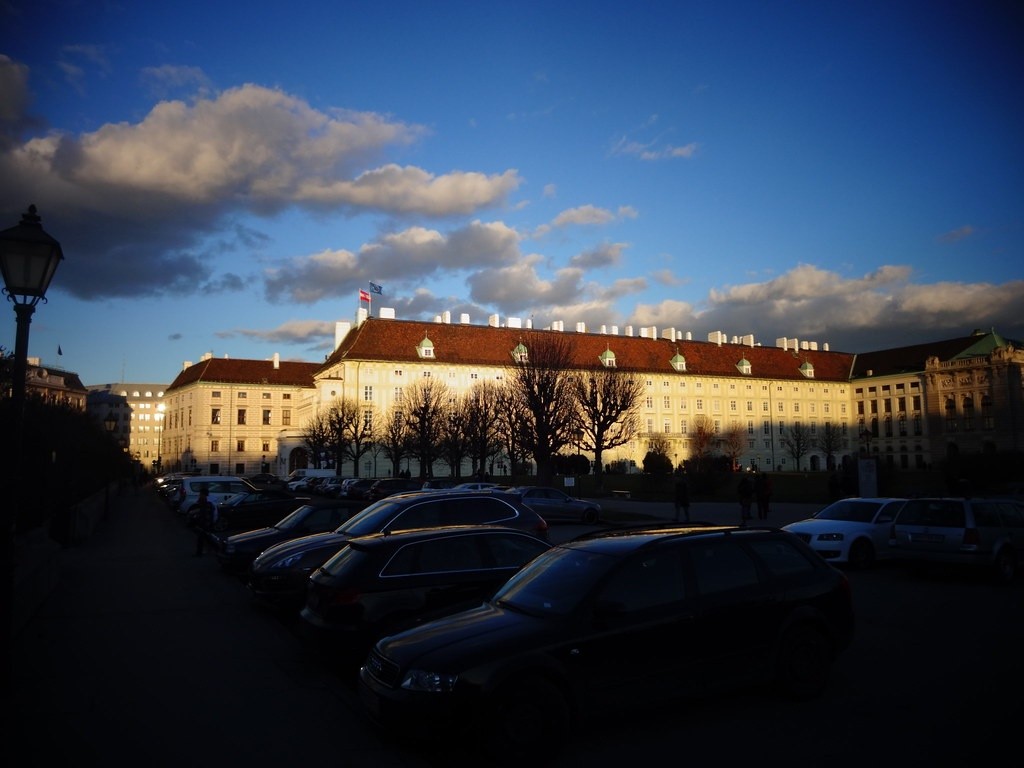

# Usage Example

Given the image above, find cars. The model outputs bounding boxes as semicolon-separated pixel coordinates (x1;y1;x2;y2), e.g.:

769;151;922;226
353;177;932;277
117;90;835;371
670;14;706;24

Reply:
159;470;364;571
780;496;908;568
290;478;603;524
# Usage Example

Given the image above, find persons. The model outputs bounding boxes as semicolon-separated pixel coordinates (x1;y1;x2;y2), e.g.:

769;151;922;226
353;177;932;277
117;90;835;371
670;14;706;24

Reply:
669;464;770;524
189;488;215;559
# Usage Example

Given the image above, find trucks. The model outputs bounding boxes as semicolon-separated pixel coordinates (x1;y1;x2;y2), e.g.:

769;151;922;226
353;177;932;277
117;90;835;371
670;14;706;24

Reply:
286;468;337;479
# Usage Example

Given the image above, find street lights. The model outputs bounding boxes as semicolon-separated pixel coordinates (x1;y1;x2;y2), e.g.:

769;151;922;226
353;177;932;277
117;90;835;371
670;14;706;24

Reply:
0;201;65;479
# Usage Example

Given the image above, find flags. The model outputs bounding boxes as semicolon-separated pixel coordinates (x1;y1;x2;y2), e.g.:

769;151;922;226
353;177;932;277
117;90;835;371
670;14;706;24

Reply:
369;282;383;295
360;288;371;301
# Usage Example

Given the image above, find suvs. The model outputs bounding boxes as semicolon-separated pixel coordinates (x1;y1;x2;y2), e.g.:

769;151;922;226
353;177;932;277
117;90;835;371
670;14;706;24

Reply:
296;517;552;648
244;487;552;614
888;496;978;566
363;525;857;734
